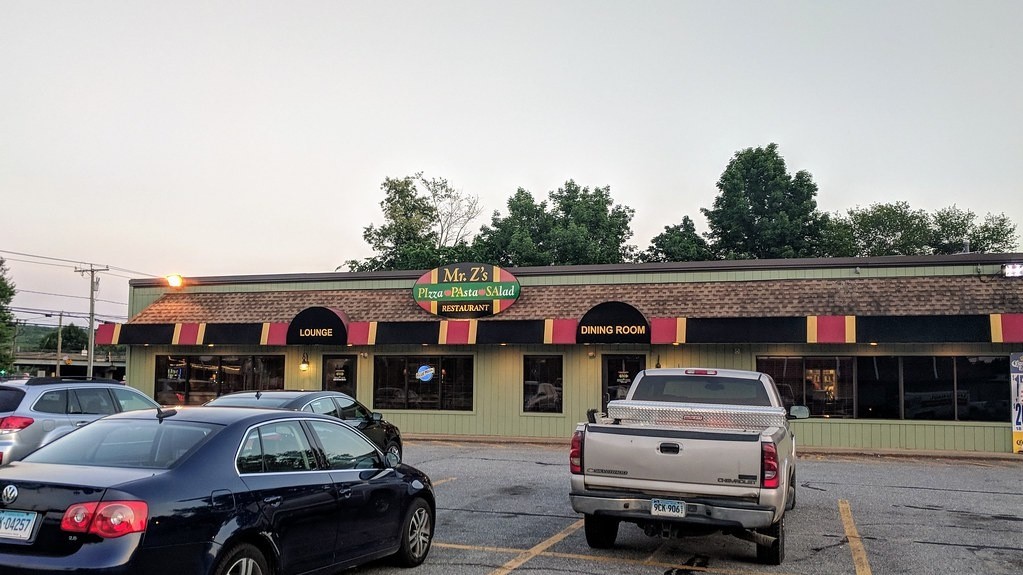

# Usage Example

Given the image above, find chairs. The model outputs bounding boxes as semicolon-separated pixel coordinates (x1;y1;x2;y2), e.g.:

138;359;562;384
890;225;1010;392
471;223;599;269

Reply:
249;439;284;471
85;398;103;413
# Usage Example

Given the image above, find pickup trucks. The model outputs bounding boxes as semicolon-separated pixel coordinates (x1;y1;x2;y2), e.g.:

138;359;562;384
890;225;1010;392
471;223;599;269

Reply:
569;367;809;565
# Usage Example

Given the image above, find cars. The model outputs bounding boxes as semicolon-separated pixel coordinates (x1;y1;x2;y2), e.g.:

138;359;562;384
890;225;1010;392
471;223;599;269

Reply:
200;389;403;462
0;407;436;575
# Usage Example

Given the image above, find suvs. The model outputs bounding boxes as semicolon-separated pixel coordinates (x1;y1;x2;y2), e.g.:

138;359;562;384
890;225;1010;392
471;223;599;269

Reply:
0;376;162;465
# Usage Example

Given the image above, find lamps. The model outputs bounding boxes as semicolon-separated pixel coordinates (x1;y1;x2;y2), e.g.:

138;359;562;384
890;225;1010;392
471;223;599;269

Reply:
299;352;310;371
